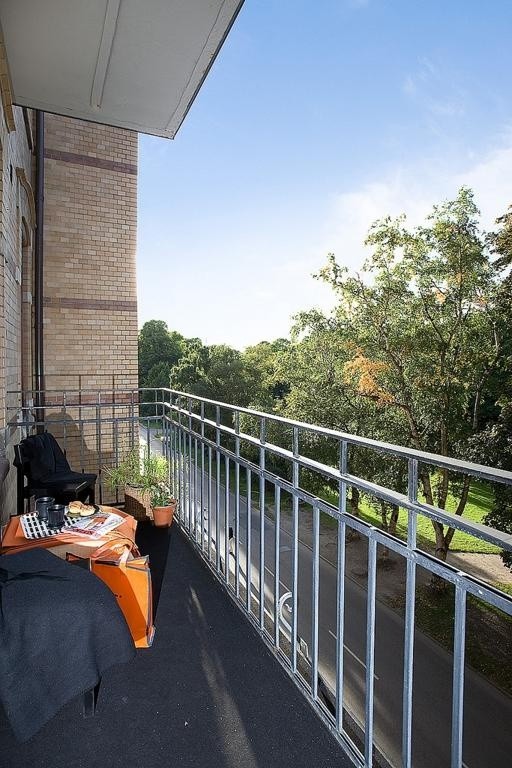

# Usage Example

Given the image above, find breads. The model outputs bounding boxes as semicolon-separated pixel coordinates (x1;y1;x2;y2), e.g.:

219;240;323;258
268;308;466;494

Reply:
80;505;95;516
69;501;82;513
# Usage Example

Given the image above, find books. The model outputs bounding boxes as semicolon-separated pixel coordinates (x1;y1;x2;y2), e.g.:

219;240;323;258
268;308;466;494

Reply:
62;513;127;539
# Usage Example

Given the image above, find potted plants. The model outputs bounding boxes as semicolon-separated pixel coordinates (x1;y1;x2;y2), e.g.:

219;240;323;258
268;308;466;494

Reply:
101;452;186;527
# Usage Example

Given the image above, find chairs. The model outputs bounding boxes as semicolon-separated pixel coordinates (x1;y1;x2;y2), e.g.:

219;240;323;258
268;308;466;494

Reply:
12;433;97;514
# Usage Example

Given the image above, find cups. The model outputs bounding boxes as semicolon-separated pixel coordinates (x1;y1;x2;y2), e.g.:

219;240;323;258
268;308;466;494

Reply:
35;497;65;530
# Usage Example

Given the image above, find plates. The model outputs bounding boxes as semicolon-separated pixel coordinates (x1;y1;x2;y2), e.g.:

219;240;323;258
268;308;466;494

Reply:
65;503;99;517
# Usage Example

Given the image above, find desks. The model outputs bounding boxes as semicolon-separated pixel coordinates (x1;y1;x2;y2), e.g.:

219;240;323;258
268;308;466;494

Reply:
8;500;138;577
